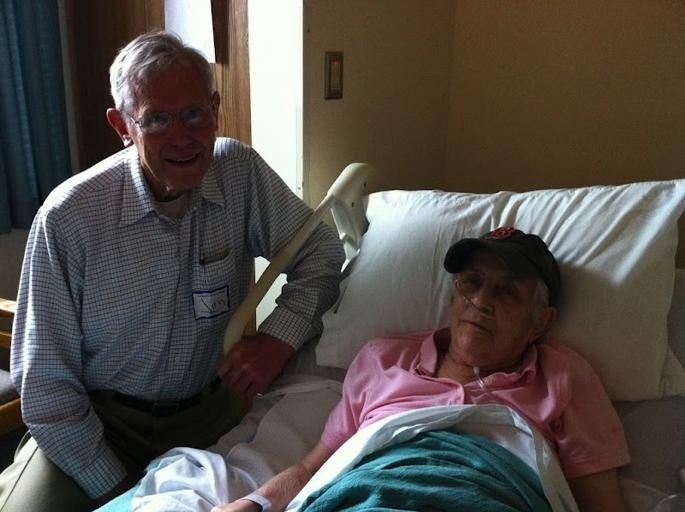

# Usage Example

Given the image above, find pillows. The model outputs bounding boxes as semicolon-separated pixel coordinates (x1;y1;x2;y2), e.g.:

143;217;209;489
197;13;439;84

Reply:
314;173;683;402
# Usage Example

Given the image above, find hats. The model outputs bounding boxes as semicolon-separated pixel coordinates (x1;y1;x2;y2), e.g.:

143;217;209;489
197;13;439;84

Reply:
444;227;560;305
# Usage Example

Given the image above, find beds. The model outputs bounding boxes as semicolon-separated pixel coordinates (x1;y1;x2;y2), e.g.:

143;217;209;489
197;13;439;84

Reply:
86;160;685;510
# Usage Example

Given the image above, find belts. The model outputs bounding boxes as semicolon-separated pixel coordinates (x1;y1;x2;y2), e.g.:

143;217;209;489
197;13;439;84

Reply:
116;381;220;417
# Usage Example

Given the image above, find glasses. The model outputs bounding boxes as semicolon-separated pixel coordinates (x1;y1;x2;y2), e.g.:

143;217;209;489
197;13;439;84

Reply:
128;104;213;136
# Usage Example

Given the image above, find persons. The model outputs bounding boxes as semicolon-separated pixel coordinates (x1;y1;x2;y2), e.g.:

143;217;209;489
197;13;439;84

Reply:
11;31;346;510
209;227;624;510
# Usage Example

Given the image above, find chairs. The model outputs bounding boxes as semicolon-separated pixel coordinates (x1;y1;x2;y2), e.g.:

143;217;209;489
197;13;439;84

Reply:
0;296;23;437
